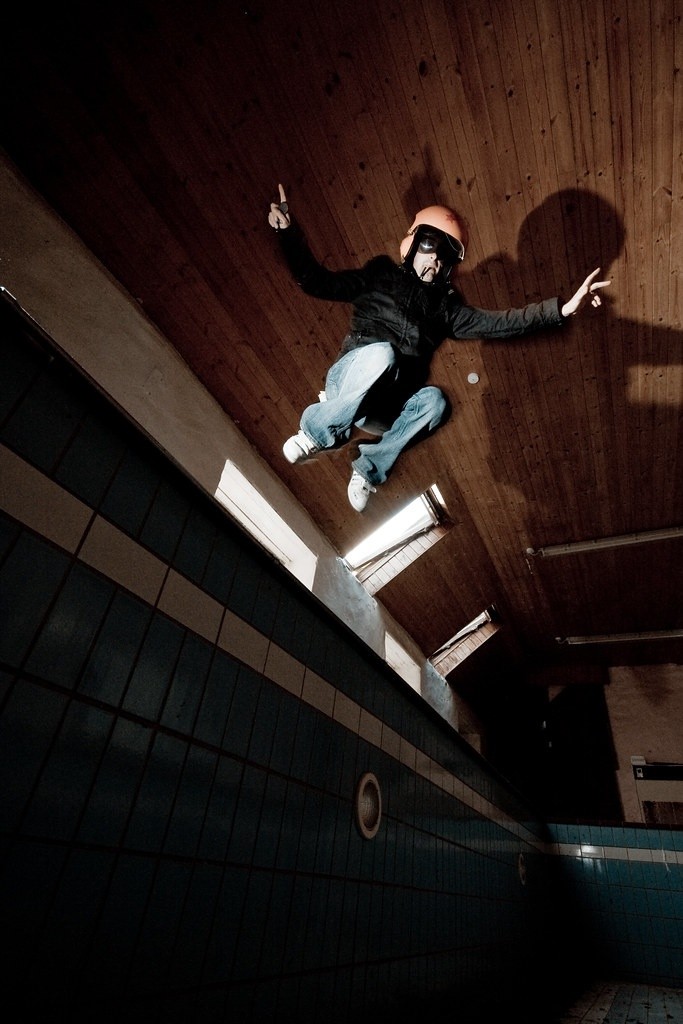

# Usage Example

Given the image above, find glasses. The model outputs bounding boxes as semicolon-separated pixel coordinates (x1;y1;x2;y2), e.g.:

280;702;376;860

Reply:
415;232;458;264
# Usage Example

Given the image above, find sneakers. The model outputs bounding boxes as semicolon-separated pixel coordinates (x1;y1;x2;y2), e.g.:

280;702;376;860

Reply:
284;433;318;465
349;472;377;514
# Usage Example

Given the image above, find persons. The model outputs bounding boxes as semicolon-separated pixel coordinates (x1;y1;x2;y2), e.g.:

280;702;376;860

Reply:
264;181;612;514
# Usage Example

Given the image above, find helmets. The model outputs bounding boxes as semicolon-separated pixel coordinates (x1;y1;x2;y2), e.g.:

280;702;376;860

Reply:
396;204;465;280
